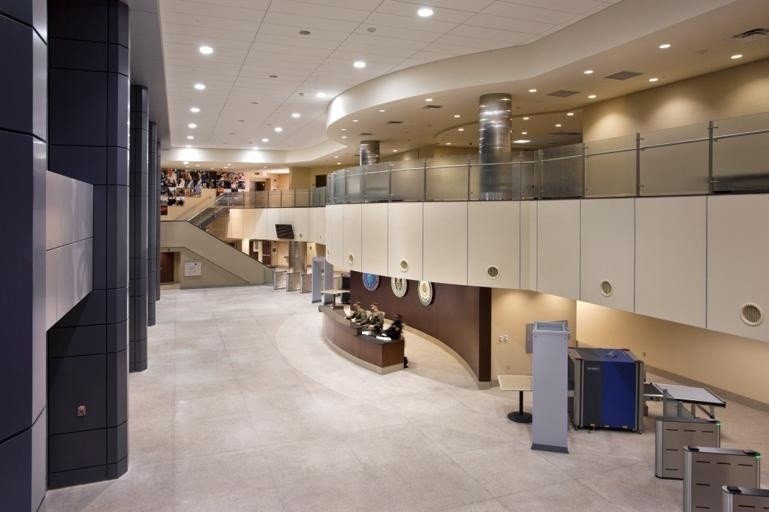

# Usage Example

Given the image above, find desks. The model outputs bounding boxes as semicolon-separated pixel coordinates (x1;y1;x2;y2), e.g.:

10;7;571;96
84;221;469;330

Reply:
320;289;350;305
497;374;532;424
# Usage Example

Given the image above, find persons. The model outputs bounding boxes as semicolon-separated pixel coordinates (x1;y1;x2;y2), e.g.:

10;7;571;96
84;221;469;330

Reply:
356;302;384;331
344;302;366;323
380;312;403;339
